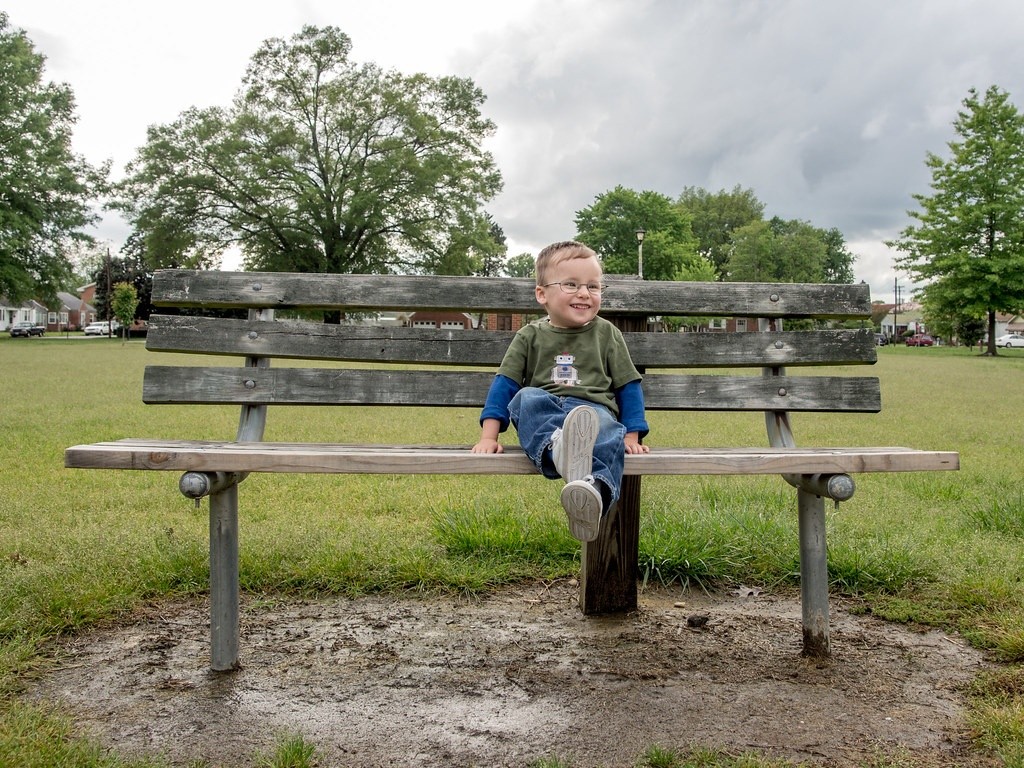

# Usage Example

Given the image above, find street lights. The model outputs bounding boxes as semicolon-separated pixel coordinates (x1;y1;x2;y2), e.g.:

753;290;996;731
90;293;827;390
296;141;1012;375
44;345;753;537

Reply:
635;228;647;279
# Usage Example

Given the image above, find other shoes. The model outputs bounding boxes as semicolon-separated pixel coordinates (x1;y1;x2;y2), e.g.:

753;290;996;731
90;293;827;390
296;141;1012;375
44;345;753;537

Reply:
561;475;603;542
548;405;599;484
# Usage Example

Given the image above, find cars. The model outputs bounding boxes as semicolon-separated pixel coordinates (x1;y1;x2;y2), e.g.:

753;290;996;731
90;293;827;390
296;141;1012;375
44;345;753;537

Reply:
905;334;933;347
874;333;888;346
995;333;1024;348
84;322;113;336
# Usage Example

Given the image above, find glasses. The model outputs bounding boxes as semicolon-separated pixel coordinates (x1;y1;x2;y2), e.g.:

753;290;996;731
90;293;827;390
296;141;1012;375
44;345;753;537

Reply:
543;278;609;295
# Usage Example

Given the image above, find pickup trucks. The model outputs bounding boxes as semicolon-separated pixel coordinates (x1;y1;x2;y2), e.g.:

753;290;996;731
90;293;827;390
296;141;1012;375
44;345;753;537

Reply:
9;321;46;338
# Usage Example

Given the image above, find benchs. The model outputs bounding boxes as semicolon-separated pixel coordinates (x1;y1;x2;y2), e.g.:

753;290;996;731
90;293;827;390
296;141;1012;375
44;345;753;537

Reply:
65;268;960;671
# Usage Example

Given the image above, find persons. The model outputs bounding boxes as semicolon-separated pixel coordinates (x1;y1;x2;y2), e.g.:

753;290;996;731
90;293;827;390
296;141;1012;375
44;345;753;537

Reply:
471;241;649;541
